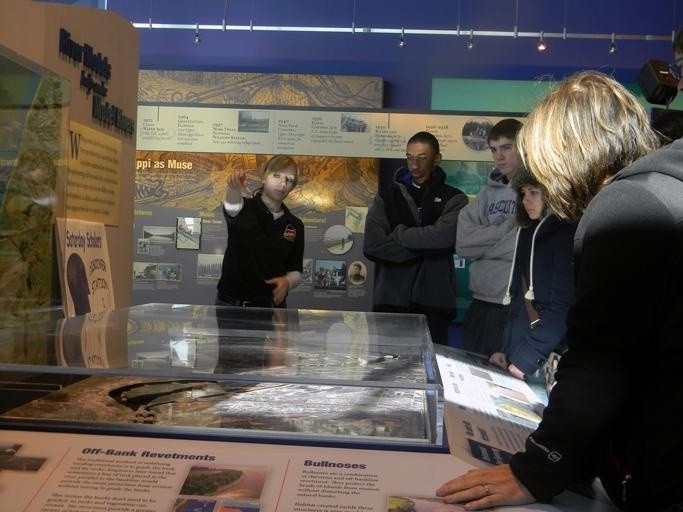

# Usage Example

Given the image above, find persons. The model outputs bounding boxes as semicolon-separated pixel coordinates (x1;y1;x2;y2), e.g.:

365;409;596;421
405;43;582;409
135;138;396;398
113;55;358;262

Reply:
437;70;682;510
491;170;608;374
670;30;683;96
363;130;468;346
316;267;340;288
456;118;535;360
349;263;366;283
214;154;304;310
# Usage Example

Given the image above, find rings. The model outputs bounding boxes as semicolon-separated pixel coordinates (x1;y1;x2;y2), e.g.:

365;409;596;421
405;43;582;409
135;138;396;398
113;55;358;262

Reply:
480;481;495;496
